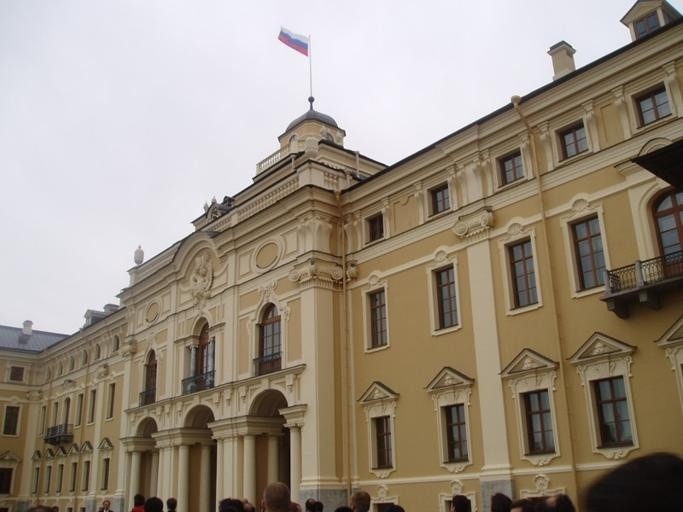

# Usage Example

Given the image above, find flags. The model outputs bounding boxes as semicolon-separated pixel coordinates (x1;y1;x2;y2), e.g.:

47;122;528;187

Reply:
279;25;310;57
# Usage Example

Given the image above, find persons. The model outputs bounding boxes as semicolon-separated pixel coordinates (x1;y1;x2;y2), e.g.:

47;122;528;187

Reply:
451;495;470;512
145;497;165;512
587;447;682;511
509;497;544;512
490;491;516;512
543;495;579;511
221;479;409;512
167;498;178;512
99;500;113;511
130;494;145;511
